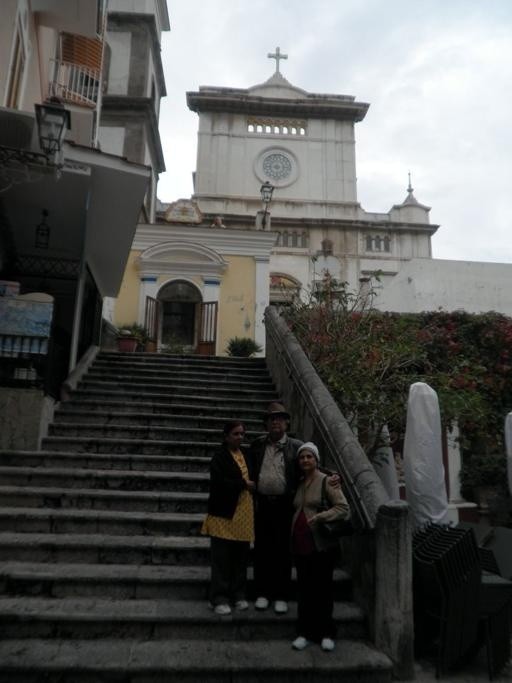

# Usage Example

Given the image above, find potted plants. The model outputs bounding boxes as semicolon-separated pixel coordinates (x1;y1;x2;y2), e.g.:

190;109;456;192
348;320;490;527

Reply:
112;323;140;352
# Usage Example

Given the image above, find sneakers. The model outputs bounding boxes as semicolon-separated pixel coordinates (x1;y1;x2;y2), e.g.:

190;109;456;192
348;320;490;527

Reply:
292;636;310;650
208;595;288;614
321;636;335;650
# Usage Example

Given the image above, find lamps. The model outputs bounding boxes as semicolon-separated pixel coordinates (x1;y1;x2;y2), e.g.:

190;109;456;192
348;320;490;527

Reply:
34;208;51;249
0;93;72;164
259;179;276;231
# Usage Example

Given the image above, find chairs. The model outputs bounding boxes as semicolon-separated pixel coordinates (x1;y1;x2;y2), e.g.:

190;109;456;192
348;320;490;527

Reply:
403;516;512;682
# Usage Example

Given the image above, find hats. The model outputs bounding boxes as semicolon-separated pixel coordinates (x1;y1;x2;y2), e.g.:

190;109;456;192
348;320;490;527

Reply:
296;441;320;462
261;402;291;417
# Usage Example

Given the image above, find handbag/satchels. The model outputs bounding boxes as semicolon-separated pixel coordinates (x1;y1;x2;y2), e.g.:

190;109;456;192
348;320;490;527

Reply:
317;503;353;557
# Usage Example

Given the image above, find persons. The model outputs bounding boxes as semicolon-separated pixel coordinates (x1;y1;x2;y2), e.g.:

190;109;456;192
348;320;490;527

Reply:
287;440;353;653
209;215;226;229
241;401;341;613
200;418;271;616
